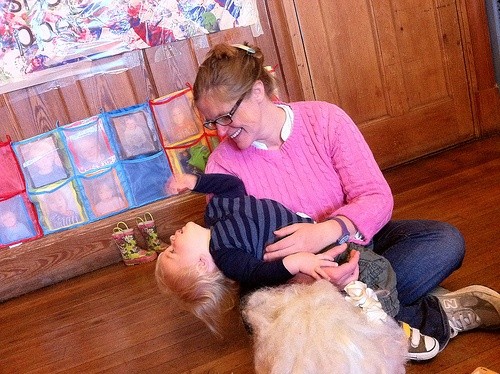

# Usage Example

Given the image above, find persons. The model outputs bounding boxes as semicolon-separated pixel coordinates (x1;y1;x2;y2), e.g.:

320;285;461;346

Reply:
72;132;108;171
43;189;82;229
167;99;198;141
29;142;65;187
90;177;124;219
0;211;35;246
152;169;441;360
191;44;500;352
180;149;202;175
121;113;154;157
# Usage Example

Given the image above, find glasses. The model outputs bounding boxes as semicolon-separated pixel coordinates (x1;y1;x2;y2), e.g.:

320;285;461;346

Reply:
202;91;251;131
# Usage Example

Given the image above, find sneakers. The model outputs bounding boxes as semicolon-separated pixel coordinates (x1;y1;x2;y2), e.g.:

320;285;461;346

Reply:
425;284;500;342
394;320;440;361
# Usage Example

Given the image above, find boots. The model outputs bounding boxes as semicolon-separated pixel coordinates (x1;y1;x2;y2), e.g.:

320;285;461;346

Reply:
111;221;158;266
135;212;170;254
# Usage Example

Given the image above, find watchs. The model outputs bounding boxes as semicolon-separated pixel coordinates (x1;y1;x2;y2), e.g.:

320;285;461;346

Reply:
321;216;351;245
